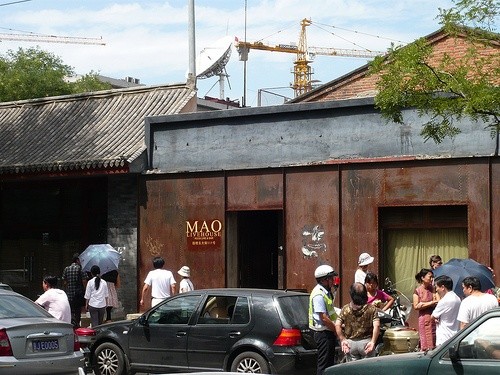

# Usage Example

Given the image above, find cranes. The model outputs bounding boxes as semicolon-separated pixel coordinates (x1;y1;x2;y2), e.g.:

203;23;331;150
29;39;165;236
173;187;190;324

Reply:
0;27;107;46
234;17;389;100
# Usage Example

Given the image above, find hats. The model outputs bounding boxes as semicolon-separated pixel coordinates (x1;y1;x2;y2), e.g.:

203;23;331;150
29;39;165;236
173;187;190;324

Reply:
177;265;191;278
358;252;375;266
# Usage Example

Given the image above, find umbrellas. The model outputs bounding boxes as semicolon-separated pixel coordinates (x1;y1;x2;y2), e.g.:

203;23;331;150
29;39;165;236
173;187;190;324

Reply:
432;258;496;301
78;243;122;276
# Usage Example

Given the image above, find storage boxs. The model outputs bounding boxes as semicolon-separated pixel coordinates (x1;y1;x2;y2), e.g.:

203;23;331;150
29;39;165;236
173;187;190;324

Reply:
383;325;420;352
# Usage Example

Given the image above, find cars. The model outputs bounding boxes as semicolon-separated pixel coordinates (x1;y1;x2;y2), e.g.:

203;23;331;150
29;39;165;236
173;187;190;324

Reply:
84;286;343;375
0;282;86;375
321;306;500;375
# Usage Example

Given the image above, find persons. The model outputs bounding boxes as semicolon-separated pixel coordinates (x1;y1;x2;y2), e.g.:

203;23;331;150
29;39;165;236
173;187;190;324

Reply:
309;264;340;375
363;272;394;311
354;252;375;285
33;273;72;324
177;265;195;294
61;253;120;328
335;281;380;363
413;255;500;359
139;256;177;309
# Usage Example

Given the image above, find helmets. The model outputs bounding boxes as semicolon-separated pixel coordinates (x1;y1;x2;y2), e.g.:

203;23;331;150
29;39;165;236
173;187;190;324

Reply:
314;264;339;280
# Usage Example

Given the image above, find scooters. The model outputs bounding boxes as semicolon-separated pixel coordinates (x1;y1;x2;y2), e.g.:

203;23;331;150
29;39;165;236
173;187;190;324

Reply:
377;276;420;356
